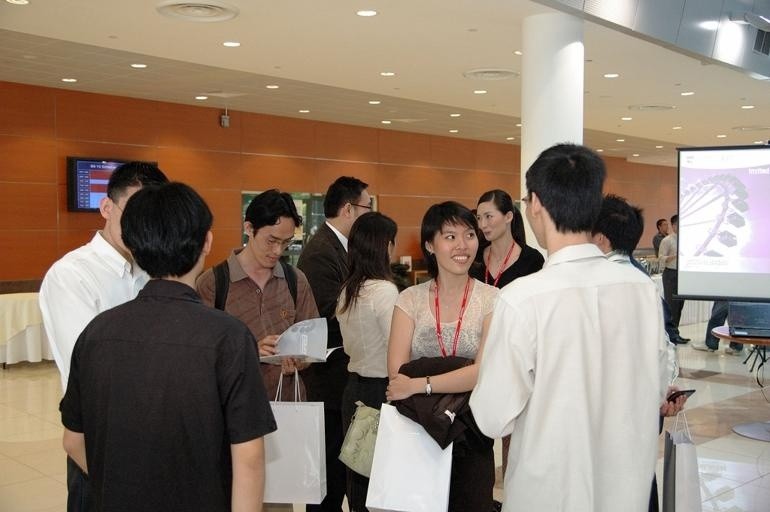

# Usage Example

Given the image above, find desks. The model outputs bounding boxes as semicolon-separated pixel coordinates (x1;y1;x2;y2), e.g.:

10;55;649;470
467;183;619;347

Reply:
411;269;433;288
647;272;714;329
1;292;56;369
710;324;770;443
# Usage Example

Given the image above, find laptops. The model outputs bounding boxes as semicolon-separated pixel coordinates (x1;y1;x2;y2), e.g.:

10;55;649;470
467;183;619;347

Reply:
728;302;770;337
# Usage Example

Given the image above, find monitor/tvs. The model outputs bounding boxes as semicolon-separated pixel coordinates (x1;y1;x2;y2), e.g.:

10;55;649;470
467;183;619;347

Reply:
66;155;158;213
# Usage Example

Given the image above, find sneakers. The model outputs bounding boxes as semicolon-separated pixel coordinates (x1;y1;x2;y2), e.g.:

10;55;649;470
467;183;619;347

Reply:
671;335;769;357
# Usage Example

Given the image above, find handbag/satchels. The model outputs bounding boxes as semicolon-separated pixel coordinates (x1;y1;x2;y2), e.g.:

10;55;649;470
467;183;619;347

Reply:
364;403;456;512
663;430;703;512
262;400;327;505
337;401;380;479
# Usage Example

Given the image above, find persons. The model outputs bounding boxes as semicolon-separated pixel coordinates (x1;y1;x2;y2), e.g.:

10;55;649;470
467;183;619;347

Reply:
34;160;171;512
469;141;669;512
195;188;322;512
651;218;669;256
334;209;404;512
591;188;697;512
467;189;545;290
295;174;374;511
384;198;505;512
659;214;693;345
691;299;744;358
60;180;280;512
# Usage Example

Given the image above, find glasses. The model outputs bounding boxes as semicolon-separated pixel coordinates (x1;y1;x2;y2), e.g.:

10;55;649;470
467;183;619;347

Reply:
252;226;295;250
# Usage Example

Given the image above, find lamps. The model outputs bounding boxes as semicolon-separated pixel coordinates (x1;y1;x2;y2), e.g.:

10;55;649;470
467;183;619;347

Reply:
728;11;770;33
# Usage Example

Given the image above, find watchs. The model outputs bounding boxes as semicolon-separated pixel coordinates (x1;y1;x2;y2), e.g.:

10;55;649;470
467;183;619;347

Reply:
424;375;432;396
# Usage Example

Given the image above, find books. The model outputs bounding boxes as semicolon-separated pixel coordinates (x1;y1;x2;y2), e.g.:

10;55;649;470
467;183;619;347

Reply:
260;316;343;367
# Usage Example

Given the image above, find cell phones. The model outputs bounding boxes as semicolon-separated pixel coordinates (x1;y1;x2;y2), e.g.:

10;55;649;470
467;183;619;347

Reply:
667;390;696;403
